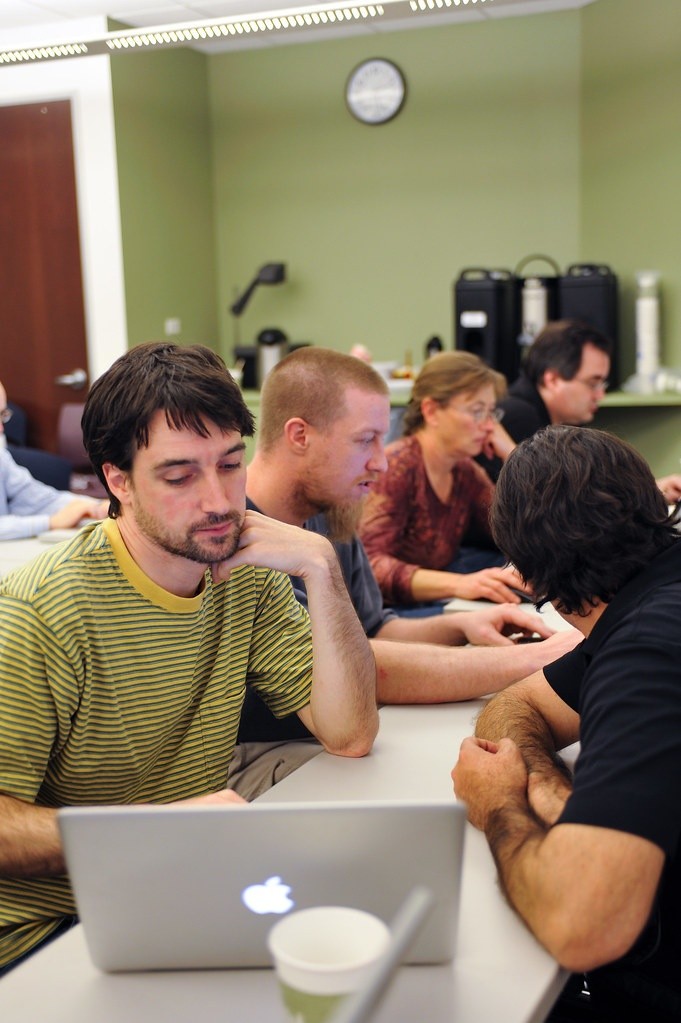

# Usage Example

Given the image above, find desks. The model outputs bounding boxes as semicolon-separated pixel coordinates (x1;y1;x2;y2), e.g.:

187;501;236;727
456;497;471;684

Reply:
0;567;569;1021
223;364;681;470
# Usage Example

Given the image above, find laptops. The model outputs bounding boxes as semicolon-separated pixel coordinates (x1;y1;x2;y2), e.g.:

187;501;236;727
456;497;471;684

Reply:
56;800;465;968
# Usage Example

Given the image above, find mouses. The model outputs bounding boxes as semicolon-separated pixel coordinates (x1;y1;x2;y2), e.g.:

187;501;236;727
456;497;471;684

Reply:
479;587;537;604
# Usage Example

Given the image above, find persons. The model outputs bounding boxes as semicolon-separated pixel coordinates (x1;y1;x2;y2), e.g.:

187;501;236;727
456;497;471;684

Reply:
357;347;545;620
227;342;595;804
0;339;380;982
448;422;681;1023
0;379;118;543
445;321;681;585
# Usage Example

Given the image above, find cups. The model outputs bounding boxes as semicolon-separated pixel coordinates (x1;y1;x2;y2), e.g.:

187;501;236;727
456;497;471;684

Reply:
266;904;390;1023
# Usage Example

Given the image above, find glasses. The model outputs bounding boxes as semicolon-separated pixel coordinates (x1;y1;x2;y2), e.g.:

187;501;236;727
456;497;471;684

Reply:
0;407;14;423
574;377;609;392
446;402;505;425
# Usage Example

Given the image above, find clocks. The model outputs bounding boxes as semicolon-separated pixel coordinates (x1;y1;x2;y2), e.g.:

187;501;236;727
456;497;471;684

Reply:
343;57;406;126
